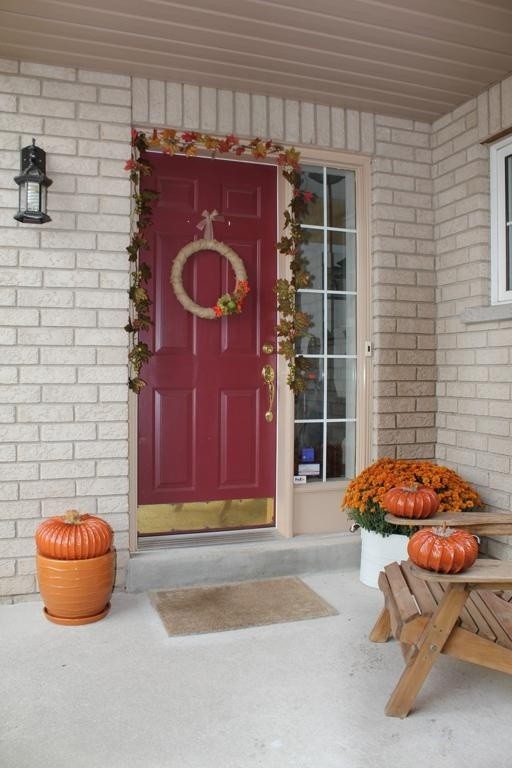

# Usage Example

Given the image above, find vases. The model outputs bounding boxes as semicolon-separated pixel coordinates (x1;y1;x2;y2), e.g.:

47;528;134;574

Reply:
360;527;410;591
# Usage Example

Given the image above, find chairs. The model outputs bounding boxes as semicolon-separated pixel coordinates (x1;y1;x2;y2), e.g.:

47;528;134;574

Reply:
370;512;512;717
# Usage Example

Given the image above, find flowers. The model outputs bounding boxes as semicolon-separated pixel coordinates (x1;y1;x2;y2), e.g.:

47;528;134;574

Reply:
341;456;481;538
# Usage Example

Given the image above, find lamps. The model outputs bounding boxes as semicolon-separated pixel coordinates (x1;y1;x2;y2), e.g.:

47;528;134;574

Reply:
13;139;52;224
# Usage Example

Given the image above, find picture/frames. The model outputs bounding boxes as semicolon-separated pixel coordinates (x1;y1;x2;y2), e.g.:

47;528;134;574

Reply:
37;546;117;626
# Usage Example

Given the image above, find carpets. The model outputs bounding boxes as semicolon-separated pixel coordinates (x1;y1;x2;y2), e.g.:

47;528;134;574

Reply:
148;576;340;638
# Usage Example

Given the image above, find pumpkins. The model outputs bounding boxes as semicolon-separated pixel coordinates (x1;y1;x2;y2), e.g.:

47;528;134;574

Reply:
384;484;440;518
36;507;112;559
408;521;480;572
